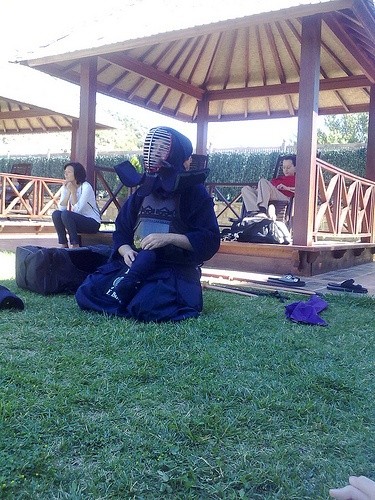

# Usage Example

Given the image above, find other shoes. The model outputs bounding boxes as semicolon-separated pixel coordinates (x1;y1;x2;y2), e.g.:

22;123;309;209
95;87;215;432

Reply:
254;213;268;218
268;204;276;221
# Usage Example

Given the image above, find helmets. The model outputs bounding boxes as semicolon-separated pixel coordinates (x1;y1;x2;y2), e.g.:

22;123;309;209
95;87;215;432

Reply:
114;126;210;197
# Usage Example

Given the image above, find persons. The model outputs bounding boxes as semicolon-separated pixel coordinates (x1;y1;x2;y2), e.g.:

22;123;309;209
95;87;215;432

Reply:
241;156;296;218
0;173;21;201
76;126;221;322
52;163;101;248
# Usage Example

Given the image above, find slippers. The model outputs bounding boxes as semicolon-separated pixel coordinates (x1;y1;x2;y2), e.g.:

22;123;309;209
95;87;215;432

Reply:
267;273;306;286
327;279;368;294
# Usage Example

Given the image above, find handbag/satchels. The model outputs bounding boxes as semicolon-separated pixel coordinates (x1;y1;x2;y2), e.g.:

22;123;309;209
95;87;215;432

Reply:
237;219;290;245
16;244;113;295
